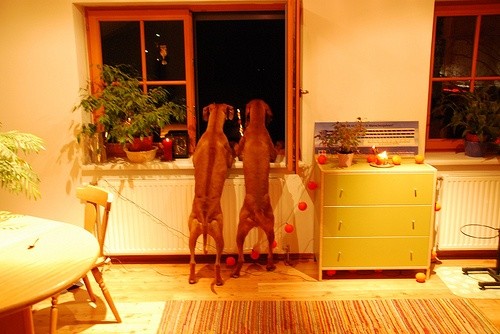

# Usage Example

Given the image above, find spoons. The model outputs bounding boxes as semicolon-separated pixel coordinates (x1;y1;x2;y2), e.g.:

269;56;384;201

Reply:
29;238;39;247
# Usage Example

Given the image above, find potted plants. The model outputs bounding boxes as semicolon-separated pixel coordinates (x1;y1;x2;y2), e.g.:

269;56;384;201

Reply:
432;77;500;156
315;118;365;166
68;60;194;160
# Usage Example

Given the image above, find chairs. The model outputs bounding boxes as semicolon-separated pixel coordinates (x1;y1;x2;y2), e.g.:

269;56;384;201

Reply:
46;185;124;334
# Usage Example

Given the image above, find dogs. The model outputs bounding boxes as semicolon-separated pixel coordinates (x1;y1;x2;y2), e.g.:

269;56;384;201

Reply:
187;102;236;286
230;97;279;280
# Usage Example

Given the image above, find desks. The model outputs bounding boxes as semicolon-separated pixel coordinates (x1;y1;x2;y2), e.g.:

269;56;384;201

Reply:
0;212;102;334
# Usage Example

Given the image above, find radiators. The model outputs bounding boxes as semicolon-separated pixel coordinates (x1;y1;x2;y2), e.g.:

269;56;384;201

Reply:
94;178;287;255
436;173;500;252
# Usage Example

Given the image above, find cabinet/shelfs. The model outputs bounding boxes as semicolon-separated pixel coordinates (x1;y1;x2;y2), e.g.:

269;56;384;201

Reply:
316;157;438;279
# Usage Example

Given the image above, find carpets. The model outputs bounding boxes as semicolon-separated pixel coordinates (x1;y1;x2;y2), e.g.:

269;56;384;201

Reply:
157;296;500;334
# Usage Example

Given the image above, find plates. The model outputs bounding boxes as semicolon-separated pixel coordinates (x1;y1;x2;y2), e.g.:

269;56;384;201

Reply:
370;162;394;168
